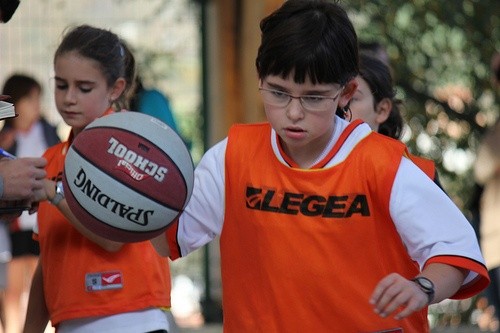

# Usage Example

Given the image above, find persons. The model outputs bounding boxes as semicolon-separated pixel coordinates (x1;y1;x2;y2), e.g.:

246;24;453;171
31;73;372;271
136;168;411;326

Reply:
0;0;192;333
92;0;490;333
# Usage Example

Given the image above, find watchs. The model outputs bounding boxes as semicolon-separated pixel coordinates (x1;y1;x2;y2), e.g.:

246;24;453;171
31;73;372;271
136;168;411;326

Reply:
411;276;435;304
50;181;64;207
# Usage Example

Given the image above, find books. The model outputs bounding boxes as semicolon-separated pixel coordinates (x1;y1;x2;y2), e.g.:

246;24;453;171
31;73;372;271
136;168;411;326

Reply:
0;94;20;120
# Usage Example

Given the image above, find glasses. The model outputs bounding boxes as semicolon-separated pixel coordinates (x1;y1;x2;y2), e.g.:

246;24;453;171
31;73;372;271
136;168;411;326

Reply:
257;76;347;112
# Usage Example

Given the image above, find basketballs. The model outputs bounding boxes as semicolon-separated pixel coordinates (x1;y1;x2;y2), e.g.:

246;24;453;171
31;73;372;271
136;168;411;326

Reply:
62;110;196;244
